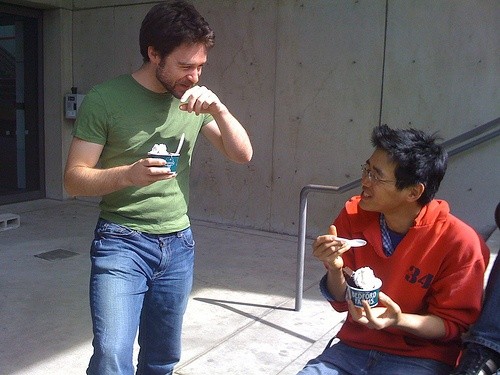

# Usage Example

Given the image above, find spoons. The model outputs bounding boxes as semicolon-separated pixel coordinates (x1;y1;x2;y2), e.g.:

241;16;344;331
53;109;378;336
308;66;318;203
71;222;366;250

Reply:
336;237;367;248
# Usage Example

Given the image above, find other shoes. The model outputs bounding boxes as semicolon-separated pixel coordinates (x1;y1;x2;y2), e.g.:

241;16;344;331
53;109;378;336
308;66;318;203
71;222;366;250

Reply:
450;344;500;375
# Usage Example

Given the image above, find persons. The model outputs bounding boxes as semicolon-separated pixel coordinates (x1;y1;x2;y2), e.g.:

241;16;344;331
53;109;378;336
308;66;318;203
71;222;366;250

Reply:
292;123;492;375
62;0;255;375
449;200;499;374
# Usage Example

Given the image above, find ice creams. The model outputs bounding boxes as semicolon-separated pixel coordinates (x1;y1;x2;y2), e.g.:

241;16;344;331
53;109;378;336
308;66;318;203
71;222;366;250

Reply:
148;143;180;174
342;266;383;308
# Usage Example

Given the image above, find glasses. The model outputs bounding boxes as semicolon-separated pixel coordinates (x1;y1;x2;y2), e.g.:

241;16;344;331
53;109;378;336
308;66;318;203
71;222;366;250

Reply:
360;164;411;185
